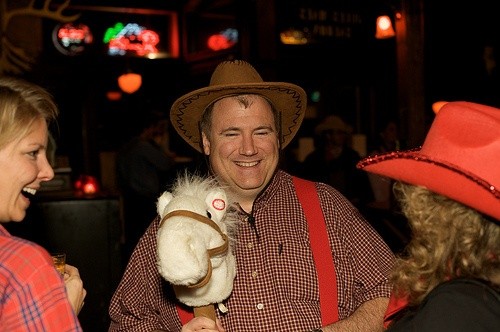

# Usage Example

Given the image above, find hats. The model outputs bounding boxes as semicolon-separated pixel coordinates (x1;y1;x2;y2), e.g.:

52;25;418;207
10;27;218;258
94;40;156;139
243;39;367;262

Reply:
314;115;353;135
357;100;500;219
168;59;307;152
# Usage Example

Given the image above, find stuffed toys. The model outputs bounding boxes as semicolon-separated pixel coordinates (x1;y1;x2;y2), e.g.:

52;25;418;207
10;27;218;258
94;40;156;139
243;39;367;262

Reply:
155;170;238;312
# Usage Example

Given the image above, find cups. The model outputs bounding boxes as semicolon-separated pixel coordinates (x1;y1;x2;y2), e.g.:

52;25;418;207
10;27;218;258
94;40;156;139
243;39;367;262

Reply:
47;253;66;276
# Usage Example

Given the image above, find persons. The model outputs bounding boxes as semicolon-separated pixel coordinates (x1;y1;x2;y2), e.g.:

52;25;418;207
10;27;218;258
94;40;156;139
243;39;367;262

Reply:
356;101;500;332
0;77;88;332
108;60;399;332
